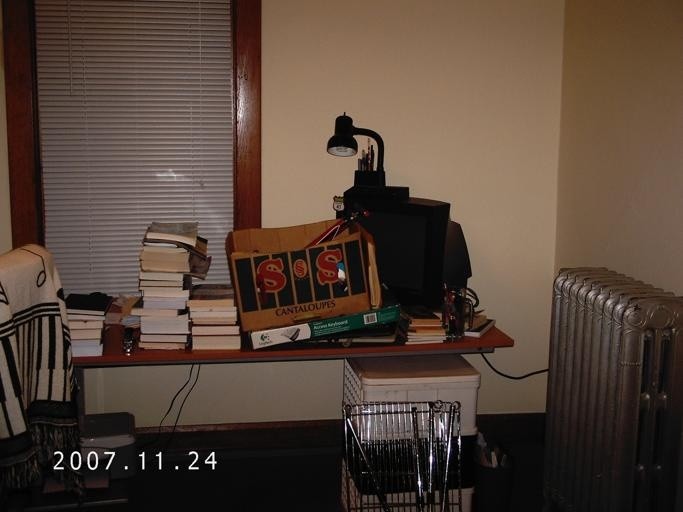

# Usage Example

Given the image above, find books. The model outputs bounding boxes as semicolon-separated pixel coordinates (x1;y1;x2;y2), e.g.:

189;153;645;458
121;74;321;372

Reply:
62;292;143;359
129;220;242;353
310;307;496;344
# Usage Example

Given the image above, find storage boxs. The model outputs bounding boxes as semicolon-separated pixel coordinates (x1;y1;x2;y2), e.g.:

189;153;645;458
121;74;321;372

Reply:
227;219;373;332
342;356;482;510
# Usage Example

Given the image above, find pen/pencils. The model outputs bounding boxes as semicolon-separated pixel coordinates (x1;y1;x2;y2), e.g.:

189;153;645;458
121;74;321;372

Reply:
444;282;448;298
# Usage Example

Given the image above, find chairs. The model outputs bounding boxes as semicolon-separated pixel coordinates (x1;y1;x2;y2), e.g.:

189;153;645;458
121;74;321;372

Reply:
0;247;139;506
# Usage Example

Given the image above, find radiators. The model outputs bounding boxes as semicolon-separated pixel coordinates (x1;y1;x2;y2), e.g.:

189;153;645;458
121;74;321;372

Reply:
544;266;681;509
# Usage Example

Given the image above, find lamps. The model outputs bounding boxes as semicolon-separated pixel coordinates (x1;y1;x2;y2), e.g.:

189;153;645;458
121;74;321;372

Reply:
325;115;387;170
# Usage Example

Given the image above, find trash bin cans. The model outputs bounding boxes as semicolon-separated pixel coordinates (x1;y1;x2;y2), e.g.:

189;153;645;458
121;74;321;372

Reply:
79;412;139;499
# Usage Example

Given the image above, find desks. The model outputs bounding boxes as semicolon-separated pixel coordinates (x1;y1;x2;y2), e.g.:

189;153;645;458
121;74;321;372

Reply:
73;324;512;437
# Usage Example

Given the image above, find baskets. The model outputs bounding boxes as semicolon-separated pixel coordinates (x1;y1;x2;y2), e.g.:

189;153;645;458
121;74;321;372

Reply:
344;400;462;511
